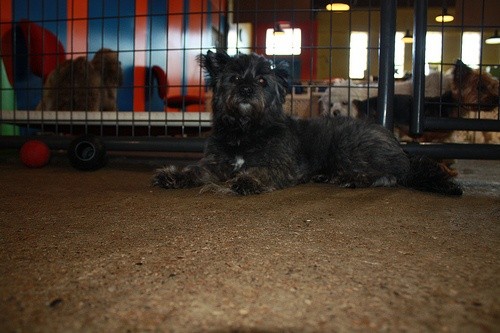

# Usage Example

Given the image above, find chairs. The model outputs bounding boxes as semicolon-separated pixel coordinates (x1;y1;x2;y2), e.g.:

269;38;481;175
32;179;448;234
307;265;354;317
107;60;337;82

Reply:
152;65;202;112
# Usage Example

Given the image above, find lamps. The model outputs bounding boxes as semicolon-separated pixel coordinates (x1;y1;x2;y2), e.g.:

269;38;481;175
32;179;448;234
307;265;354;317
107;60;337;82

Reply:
485;29;500;45
325;0;351;11
435;6;454;22
404;29;413;38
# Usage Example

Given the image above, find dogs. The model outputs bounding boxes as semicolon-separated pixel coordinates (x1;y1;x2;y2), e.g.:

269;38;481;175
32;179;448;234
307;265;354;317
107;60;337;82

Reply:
152;50;431;197
318;58;499;144
33;49;123;136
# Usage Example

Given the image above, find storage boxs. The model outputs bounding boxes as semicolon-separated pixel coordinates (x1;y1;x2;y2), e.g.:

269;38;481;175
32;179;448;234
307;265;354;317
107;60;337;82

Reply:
283;92;321;119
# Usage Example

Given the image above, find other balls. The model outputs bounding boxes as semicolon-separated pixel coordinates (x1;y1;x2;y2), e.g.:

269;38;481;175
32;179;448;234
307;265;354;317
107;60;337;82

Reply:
21;141;48;166
